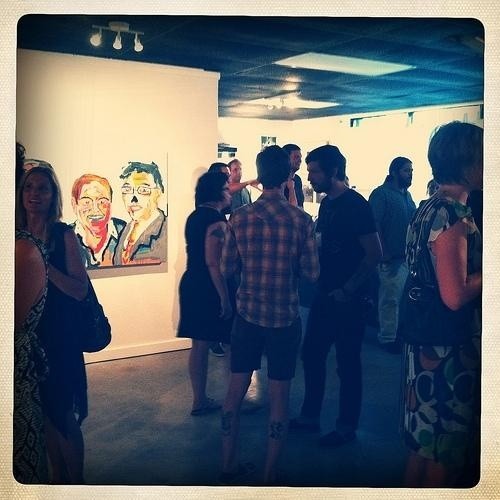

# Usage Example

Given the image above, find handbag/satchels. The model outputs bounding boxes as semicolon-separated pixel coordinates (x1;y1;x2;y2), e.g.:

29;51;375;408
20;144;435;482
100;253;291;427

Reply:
396;266;473;347
54;222;111;352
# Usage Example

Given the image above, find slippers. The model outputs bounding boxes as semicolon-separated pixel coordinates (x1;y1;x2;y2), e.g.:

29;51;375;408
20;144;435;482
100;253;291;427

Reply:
191;398;222;416
327;430;356;446
288;418;321;431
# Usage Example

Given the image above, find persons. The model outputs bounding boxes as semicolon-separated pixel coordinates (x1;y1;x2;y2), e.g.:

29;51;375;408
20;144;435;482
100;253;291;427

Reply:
21;166;114;483
16;143;53;483
175;121;482;486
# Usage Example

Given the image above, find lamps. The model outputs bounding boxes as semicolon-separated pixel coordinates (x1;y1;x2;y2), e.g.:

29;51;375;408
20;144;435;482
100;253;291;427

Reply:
86;21;145;55
348;104;484;128
